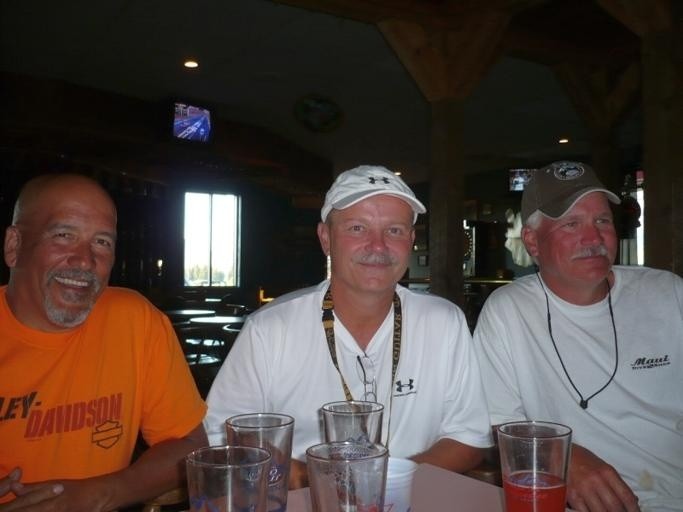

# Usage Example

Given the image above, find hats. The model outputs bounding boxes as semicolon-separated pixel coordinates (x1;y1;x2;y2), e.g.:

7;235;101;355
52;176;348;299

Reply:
321;164;427;227
521;161;622;227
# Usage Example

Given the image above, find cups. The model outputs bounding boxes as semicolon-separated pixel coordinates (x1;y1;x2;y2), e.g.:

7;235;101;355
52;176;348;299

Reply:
303;399;420;512
493;421;573;512
184;410;295;512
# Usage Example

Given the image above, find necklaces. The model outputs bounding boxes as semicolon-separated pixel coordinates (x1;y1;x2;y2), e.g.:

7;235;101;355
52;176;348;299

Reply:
535;267;619;409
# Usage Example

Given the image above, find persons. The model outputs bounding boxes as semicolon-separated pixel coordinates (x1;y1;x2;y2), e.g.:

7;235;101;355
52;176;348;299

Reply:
0;172;211;511
474;161;682;512
200;164;497;490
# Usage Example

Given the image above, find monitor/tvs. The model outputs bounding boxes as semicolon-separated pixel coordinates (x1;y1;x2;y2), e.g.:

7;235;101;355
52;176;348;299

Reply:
167;99;212;144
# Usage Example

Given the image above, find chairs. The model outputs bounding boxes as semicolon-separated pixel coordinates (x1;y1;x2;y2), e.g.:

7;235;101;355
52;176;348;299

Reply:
164;304;246;371
